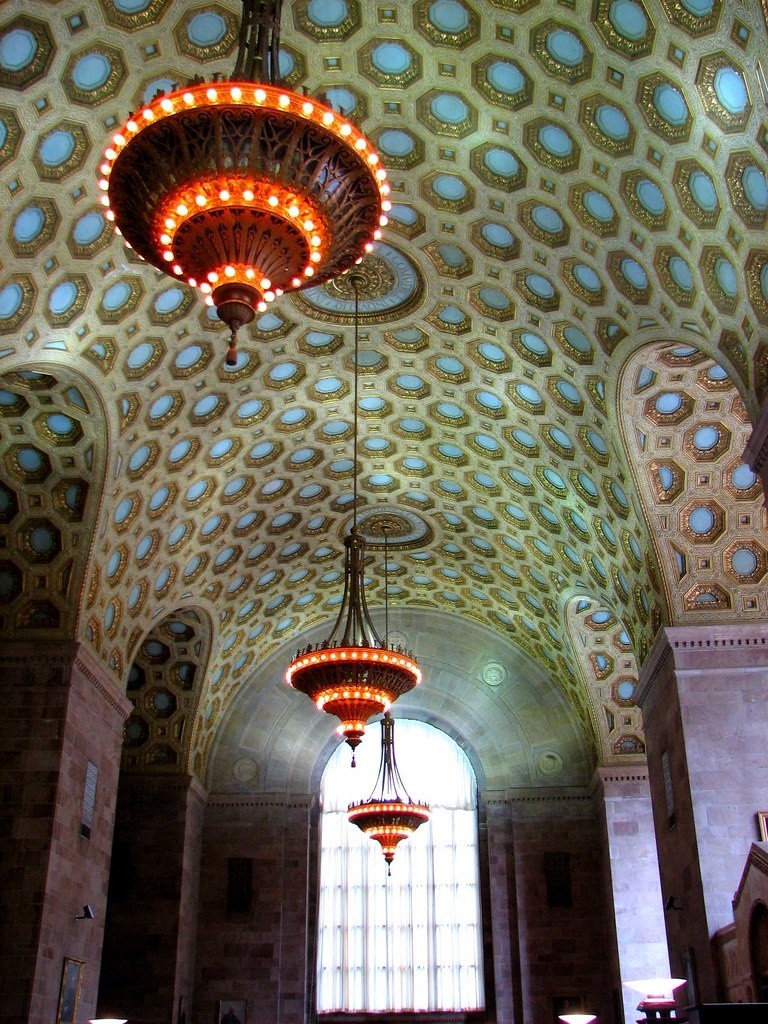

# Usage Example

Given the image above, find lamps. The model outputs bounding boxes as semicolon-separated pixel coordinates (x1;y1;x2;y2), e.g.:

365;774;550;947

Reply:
76;904;94;920
664;897;685;911
286;273;424;770
346;711;430;877
94;1;393;366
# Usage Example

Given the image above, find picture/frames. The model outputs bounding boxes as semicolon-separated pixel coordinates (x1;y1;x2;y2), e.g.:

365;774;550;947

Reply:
218;1000;249;1024
549;994;584;1024
57;956;86;1024
178;995;192;1024
